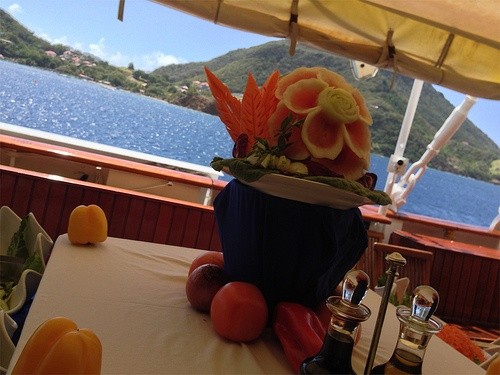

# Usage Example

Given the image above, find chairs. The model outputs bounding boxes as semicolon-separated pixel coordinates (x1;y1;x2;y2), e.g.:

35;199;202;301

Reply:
374;243;433;303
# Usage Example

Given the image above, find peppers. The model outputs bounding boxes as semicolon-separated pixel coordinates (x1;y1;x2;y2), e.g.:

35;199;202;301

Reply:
11;317;102;375
68;204;108;244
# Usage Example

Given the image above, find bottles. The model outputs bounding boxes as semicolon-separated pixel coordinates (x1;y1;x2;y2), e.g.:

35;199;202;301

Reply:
299;269;372;374
370;285;444;375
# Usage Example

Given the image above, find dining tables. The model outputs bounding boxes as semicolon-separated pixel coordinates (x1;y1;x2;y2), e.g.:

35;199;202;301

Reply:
9;233;484;375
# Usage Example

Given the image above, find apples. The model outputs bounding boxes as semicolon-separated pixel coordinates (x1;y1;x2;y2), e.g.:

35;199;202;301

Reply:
186;251;267;341
